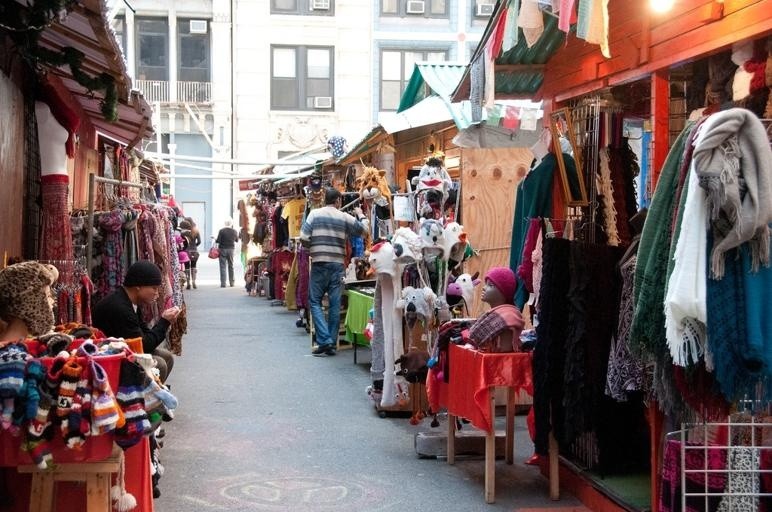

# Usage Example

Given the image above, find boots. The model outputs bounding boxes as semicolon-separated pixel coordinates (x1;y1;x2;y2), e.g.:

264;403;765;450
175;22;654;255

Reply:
184;268;198;289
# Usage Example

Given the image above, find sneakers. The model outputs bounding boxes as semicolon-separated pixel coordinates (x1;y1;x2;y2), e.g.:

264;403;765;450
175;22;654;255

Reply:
220;283;234;288
313;344;335;356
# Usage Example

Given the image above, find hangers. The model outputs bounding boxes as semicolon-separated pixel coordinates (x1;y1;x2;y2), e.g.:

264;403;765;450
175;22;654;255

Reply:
70;178;144;217
543;199;609;241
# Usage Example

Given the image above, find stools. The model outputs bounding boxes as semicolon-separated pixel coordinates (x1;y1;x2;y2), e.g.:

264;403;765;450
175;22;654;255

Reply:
17;455;123;512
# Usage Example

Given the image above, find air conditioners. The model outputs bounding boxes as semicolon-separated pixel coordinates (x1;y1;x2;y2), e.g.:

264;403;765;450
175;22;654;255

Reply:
312;0;330;10
314;97;332;109
475;4;494;17
190;20;207;34
406;1;425;14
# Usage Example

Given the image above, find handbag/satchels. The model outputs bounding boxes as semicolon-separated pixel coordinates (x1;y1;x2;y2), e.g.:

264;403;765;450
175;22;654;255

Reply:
208;247;220;259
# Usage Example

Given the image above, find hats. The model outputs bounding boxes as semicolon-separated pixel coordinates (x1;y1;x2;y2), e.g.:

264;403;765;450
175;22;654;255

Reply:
391;159;476;383
1;261;60;335
485;269;515;301
124;260;162;287
178;251;190;262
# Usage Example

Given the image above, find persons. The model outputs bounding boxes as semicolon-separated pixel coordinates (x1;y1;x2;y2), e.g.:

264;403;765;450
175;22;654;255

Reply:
178;215;202;290
33;100;74;261
211;215;239;288
467;266;524;353
0;258;61;344
89;259;183;438
239;226;251;273
298;186;370;356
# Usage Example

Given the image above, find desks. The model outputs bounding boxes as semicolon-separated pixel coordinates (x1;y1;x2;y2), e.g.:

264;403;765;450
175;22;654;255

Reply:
343;288;374;365
9;434;154;512
425;340;560;504
251;258;267;296
